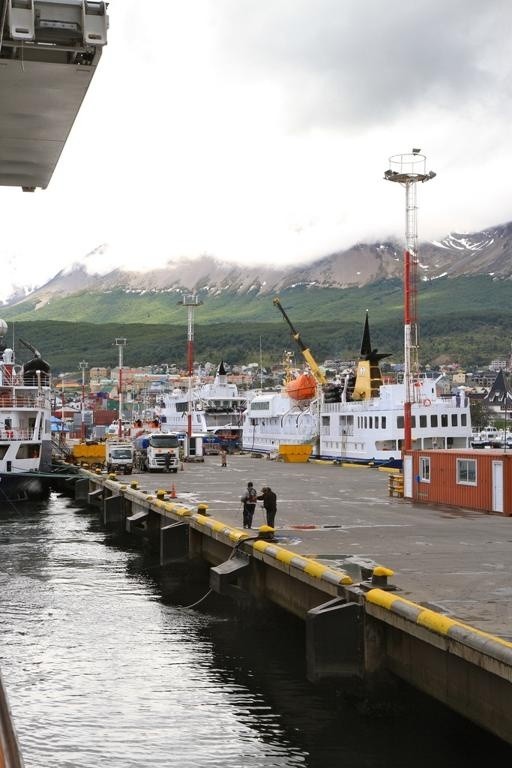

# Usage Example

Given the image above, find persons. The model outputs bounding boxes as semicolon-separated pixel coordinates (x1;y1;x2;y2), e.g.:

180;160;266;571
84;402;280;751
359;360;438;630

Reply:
255;487;277;529
219;449;228;467
240;482;257;530
165;452;171;473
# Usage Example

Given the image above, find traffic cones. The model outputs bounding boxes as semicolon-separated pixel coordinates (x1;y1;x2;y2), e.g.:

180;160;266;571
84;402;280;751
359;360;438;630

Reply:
169;481;177;498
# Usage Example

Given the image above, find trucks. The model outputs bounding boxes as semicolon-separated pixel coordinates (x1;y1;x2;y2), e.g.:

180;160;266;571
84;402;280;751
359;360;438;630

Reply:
132;433;181;473
105;437;134;474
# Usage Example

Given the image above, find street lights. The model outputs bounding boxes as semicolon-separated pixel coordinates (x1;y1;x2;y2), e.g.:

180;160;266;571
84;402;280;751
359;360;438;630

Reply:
383;146;437;452
112;336;128;438
79;359;88;442
176;292;203;436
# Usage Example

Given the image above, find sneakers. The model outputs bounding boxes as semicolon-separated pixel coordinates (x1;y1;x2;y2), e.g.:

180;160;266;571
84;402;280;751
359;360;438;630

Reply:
243;523;252;529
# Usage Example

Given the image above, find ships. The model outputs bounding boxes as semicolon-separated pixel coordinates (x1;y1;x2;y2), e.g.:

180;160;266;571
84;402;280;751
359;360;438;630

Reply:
0;317;53;510
159;359;247;433
242;366;472;463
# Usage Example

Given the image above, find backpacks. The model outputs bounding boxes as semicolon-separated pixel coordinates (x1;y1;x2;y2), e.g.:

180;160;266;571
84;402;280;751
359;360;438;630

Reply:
247;488;257;502
